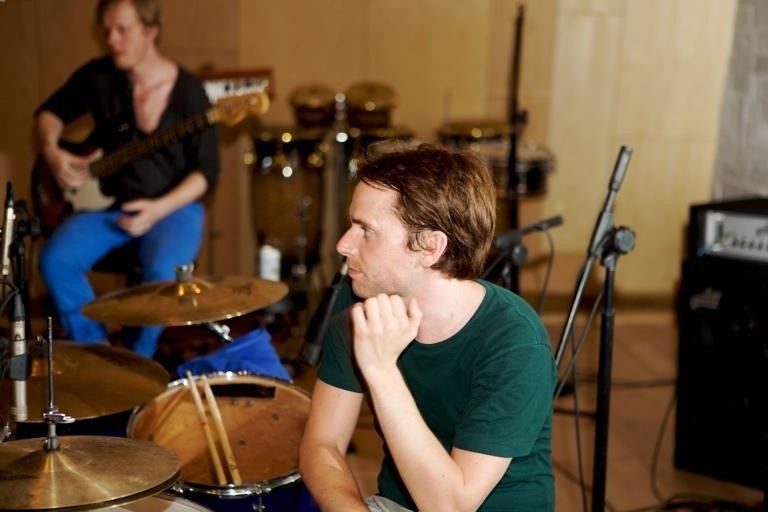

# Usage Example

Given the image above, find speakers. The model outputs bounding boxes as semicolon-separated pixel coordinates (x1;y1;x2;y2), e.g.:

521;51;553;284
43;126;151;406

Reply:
672;194;768;494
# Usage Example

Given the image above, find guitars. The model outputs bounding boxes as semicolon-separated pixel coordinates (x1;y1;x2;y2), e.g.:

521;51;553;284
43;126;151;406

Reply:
30;90;271;228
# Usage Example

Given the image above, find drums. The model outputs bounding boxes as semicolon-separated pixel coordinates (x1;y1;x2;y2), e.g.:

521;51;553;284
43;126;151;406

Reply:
124;369;320;512
474;147;550;199
438;116;511;151
288;84;336;129
344;81;398;131
250;123;415;172
56;487;218;512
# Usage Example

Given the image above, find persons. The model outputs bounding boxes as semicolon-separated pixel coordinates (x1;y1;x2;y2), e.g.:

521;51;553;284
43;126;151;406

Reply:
297;141;557;512
35;1;217;358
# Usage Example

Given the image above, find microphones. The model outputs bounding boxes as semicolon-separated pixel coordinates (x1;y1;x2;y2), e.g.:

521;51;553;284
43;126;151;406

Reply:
9;294;29;423
300;272;345;369
0;192;17;277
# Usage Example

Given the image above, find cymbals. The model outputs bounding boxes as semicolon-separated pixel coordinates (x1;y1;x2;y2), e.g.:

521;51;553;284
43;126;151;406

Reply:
0;434;181;512
0;336;171;427
81;273;291;330
